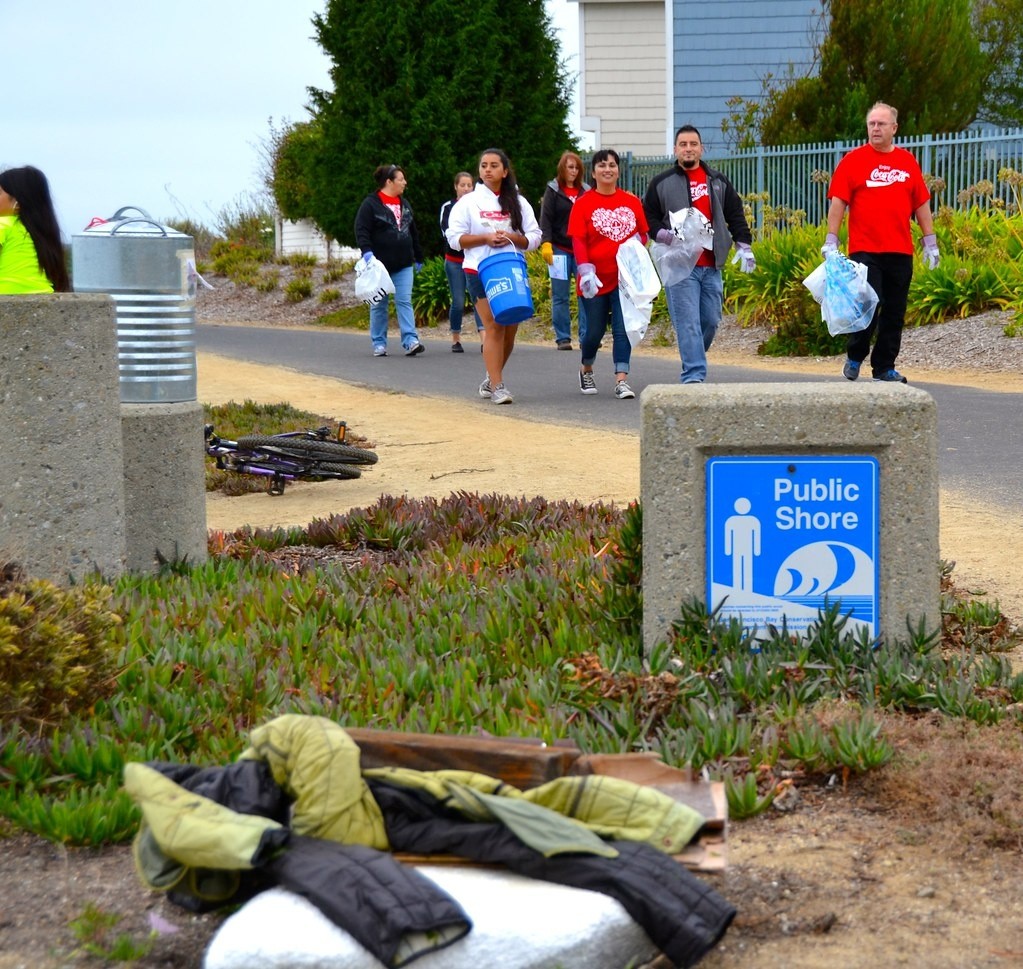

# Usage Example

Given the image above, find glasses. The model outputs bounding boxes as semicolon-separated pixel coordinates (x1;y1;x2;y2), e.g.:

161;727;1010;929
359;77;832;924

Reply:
387;164;396;177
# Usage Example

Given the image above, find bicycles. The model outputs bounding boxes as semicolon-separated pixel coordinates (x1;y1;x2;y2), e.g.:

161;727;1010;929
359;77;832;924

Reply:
204;419;380;497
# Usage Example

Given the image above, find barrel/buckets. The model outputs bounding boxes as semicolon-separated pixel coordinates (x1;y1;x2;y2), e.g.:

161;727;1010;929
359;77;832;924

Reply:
478;235;535;325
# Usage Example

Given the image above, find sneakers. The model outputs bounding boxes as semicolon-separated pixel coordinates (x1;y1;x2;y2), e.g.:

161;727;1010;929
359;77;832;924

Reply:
872;367;908;383
843;359;862;380
479;370;492;398
614;380;635;399
406;342;424;356
578;370;597;395
373;346;388;356
491;383;512;404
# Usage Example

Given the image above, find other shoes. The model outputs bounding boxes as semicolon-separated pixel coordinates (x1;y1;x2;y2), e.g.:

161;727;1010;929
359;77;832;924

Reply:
452;341;464;352
481;345;483;352
580;342;602;349
557;340;573;350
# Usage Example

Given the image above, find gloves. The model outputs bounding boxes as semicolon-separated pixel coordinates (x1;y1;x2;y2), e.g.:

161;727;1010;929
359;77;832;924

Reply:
731;242;756;273
363;251;373;263
655;228;672;247
542;242;554;266
821;234;841;259
918;234;939;270
416;263;422;272
576;263;603;298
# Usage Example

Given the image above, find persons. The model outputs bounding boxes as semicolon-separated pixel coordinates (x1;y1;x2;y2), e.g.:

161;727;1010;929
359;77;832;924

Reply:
440;172;486;353
541;153;602;350
644;124;756;383
821;104;940;384
357;167;426;356
0;166;69;294
445;150;543;405
568;150;649;398
